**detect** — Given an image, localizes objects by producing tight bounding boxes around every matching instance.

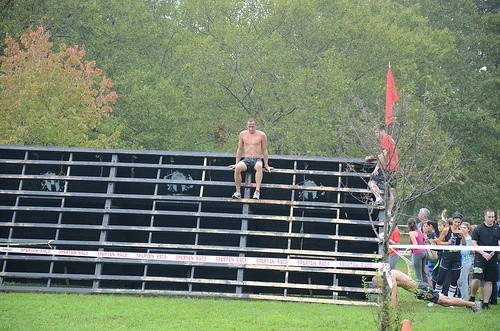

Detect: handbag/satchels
[431,251,438,263]
[424,242,432,259]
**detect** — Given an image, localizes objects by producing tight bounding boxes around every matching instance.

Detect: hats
[452,213,463,218]
[430,221,437,227]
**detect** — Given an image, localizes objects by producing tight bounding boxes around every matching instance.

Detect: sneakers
[253,190,260,199]
[232,192,241,199]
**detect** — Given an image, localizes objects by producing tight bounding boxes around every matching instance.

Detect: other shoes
[482,303,492,310]
[469,296,475,302]
[373,197,383,206]
[386,209,391,215]
[472,301,482,314]
[490,301,497,305]
[427,302,435,307]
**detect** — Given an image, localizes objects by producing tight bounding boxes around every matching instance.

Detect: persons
[228,119,274,200]
[372,208,500,313]
[366,123,398,216]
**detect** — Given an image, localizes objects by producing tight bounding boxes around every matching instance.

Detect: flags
[386,66,397,127]
